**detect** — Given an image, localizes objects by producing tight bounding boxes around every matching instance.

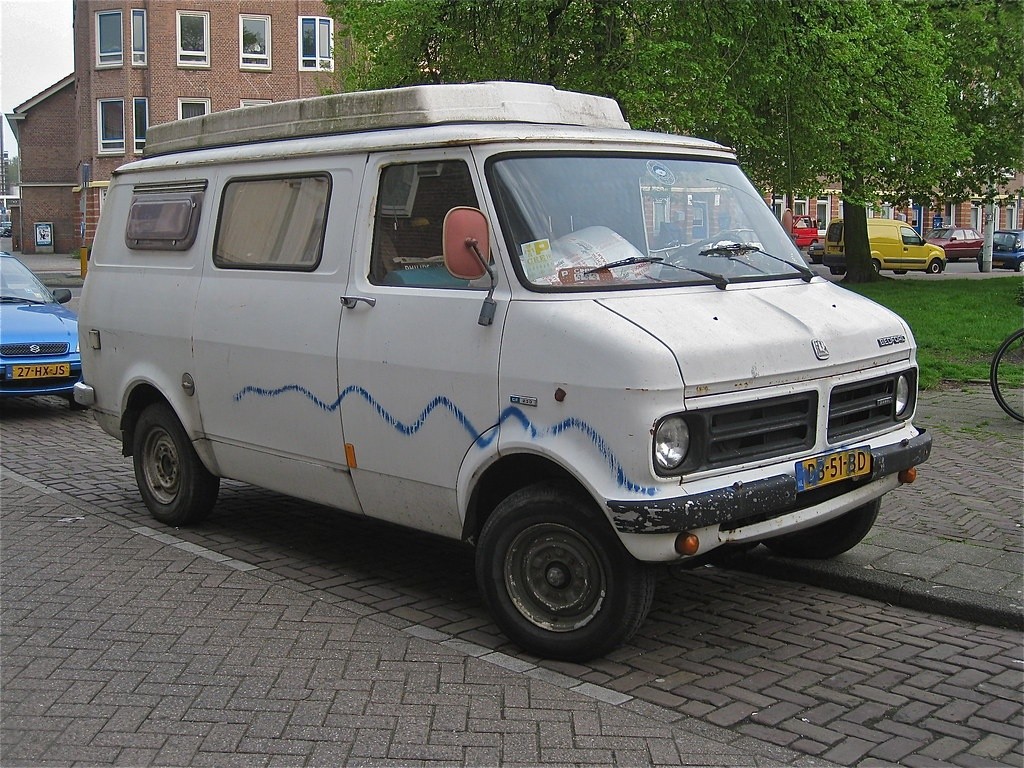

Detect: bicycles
[991,330,1024,422]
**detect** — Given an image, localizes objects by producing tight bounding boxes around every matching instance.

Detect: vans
[74,80,933,663]
[790,215,947,275]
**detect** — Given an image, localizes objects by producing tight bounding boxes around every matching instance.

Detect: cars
[923,228,984,262]
[977,230,1024,272]
[0,252,88,411]
